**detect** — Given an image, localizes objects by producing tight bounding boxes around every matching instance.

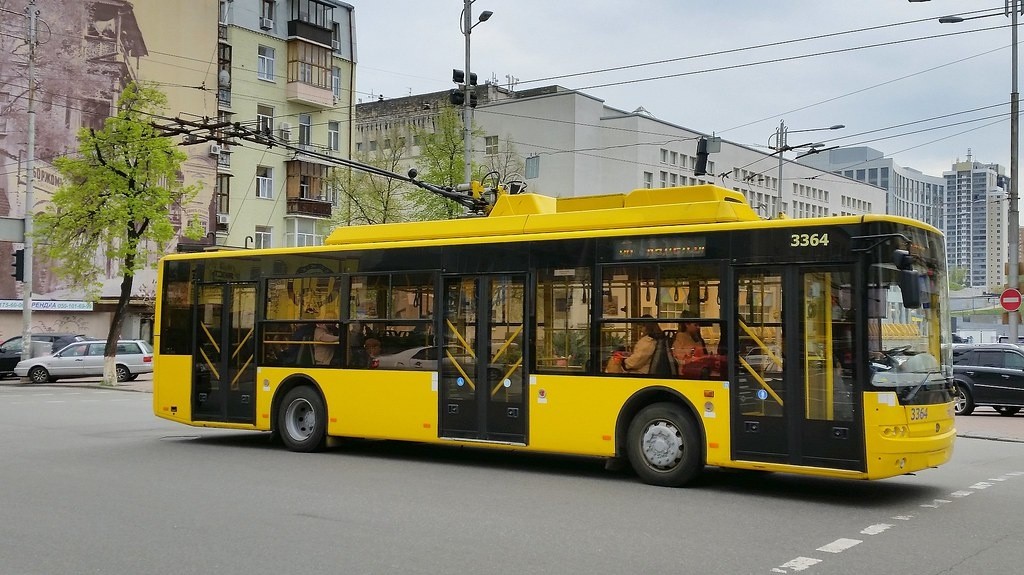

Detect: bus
[941,343,1024,416]
[152,122,958,481]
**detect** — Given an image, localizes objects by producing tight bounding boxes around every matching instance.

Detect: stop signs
[1000,288,1023,312]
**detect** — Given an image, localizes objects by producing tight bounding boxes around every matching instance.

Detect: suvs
[0,332,94,381]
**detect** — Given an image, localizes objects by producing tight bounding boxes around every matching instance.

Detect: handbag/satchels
[605,354,628,373]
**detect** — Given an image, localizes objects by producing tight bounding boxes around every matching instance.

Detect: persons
[406,315,430,345]
[615,314,662,373]
[272,308,371,363]
[838,311,884,379]
[359,340,382,368]
[670,309,760,378]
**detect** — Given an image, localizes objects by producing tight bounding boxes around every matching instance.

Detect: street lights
[938,0,1019,344]
[777,118,845,218]
[464,0,494,216]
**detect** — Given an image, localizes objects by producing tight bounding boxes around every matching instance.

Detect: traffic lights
[11,249,23,282]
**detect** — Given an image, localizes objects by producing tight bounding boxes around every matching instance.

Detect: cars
[14,339,153,384]
[953,335,1024,376]
[367,339,946,411]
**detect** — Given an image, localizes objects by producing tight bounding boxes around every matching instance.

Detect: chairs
[292,326,318,367]
[650,329,704,376]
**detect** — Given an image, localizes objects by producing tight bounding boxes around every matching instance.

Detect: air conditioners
[262,18,273,29]
[210,145,220,154]
[332,40,340,50]
[333,95,338,105]
[280,122,291,131]
[281,131,291,141]
[216,214,230,225]
